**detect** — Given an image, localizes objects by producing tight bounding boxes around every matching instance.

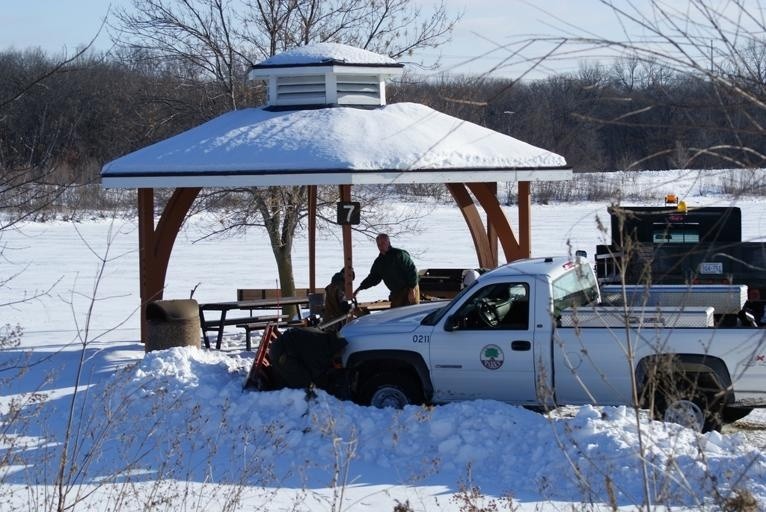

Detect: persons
[354,234,420,308]
[325,268,355,322]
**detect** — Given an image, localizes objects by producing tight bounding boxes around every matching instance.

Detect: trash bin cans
[145,300,202,352]
[309,292,324,320]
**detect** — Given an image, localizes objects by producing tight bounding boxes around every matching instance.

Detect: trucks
[574,205,766,300]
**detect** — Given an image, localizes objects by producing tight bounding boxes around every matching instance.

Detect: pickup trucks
[337,258,766,433]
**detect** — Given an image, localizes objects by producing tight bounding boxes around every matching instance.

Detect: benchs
[236,322,289,351]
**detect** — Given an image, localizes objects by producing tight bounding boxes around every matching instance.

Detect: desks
[200,299,310,348]
